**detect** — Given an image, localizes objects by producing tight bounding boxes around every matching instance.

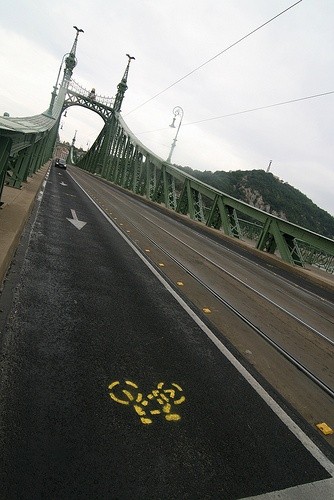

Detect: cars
[54,157,67,170]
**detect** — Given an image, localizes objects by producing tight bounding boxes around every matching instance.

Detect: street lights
[42,52,79,120]
[163,106,184,162]
